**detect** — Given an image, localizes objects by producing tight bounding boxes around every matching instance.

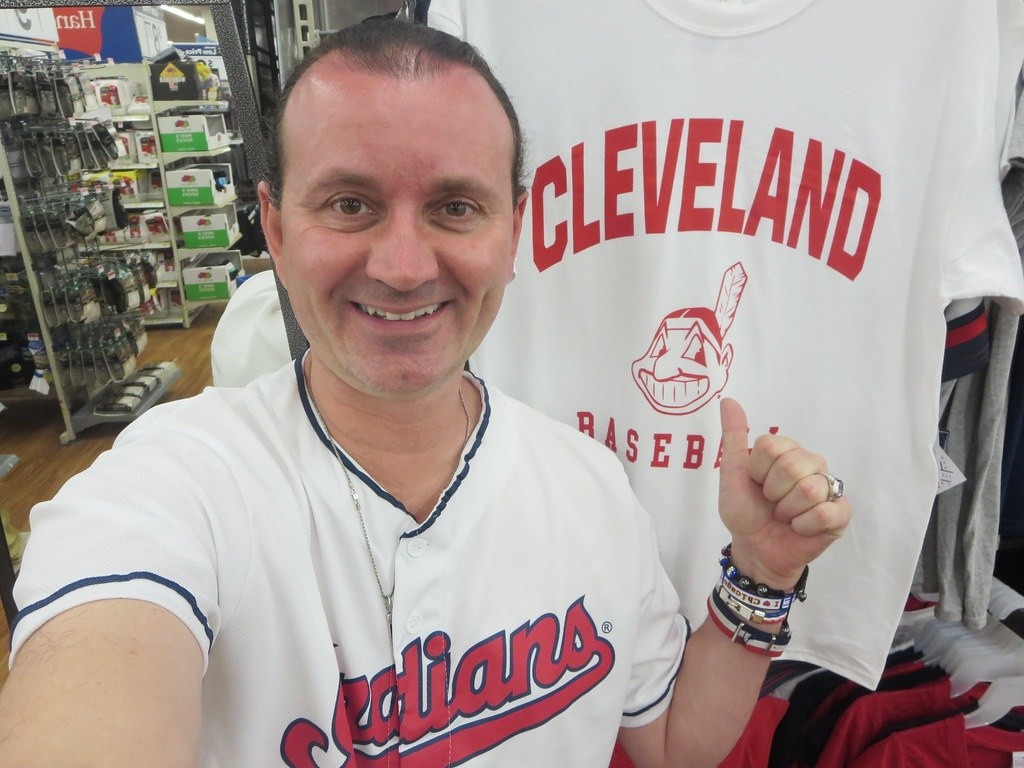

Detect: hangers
[898,575,1024,728]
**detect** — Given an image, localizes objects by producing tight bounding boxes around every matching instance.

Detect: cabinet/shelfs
[0,39,242,445]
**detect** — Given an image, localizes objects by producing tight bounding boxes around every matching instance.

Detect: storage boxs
[158,114,228,152]
[165,163,235,206]
[179,204,238,249]
[182,250,241,300]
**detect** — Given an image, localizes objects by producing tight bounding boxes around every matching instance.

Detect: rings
[816,472,844,502]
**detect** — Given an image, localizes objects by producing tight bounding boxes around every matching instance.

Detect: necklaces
[306,387,470,641]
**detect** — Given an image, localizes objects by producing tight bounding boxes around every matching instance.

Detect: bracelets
[707,543,809,658]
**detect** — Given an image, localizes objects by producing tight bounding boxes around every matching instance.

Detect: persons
[0,18,851,768]
